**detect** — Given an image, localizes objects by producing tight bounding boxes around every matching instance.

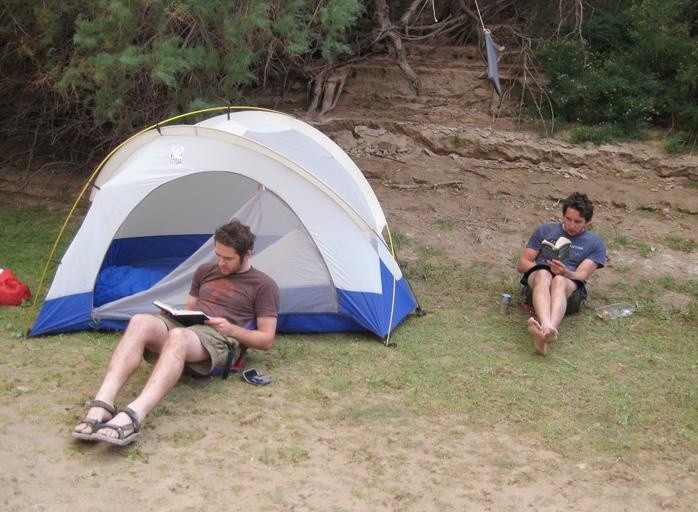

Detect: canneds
[499,293,511,314]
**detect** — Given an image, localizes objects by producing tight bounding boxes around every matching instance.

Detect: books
[540,237,572,262]
[151,301,210,327]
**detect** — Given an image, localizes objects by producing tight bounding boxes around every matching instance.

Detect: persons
[70,217,280,445]
[515,191,607,356]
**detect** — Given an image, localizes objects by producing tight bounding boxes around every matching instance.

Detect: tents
[21,103,430,353]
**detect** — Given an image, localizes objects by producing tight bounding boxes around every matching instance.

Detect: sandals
[72,400,118,442]
[96,407,142,446]
